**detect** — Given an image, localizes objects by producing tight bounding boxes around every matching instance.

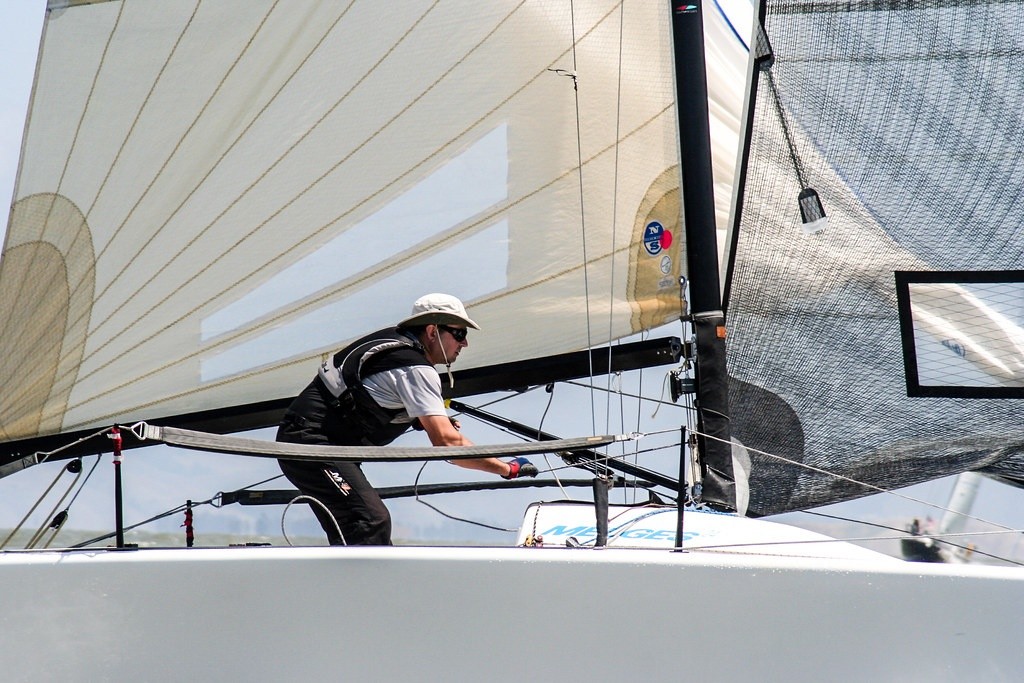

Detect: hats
[397,293,481,330]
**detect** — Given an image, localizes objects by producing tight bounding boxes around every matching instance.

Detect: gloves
[500,457,538,480]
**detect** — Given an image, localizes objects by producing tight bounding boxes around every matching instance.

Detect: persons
[273,289,538,550]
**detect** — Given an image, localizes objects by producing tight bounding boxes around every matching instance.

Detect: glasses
[438,324,467,342]
